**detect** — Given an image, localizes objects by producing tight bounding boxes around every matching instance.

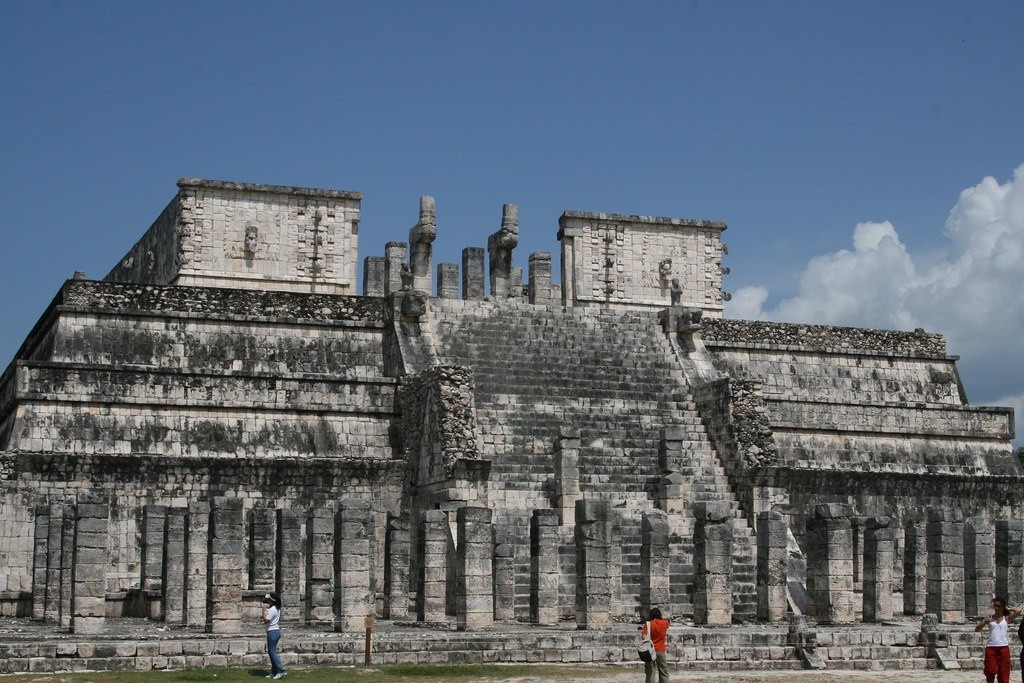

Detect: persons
[261,593,288,680]
[641,607,670,683]
[975,598,1021,683]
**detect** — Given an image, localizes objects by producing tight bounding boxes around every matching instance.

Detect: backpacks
[638,622,657,662]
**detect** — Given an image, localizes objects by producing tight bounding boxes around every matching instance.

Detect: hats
[265,594,277,602]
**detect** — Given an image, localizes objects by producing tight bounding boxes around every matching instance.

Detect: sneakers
[265,672,275,678]
[272,672,287,680]
